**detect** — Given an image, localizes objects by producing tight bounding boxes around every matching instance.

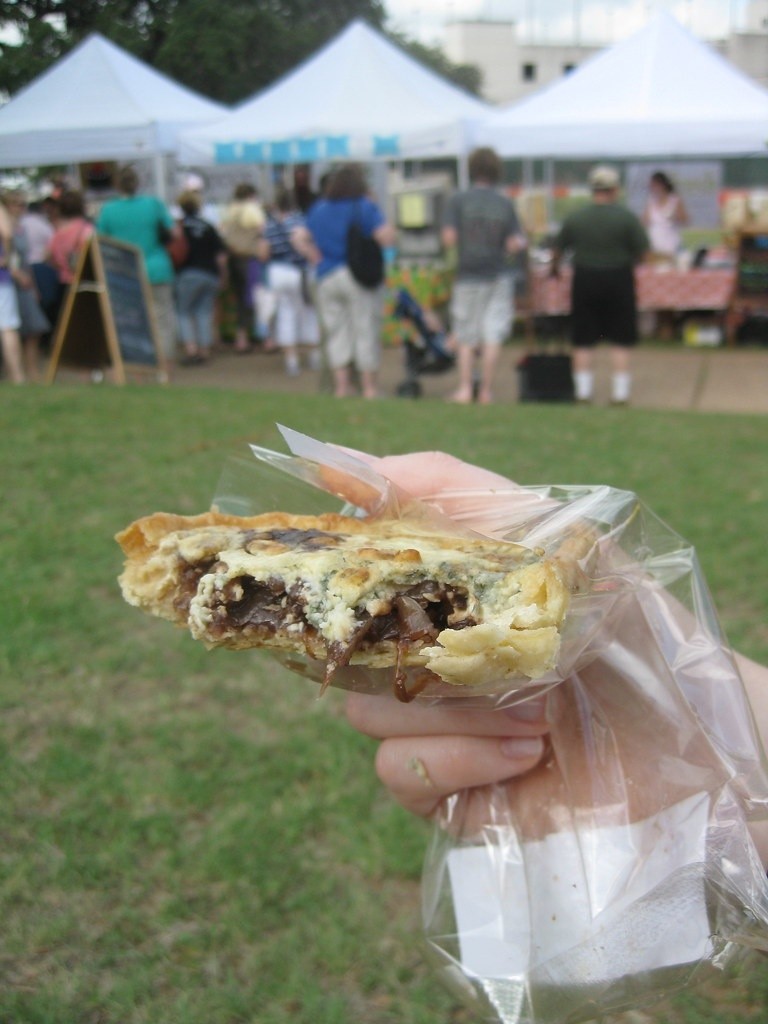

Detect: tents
[481,15,768,182]
[177,23,489,195]
[1,34,232,194]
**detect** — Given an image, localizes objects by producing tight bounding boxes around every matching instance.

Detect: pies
[104,510,566,700]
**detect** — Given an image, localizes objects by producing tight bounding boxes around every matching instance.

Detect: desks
[514,264,737,340]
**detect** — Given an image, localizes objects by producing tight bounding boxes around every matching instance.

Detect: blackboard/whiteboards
[53,235,169,371]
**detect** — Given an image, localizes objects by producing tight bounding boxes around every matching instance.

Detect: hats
[590,167,617,189]
[183,173,203,191]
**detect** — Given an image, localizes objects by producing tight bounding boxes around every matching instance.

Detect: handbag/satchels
[348,225,384,287]
[519,353,572,401]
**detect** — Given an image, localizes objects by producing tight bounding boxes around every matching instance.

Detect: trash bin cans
[517,352,579,402]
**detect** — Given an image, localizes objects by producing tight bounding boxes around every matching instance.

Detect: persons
[646,173,690,338]
[0,164,326,389]
[314,444,768,870]
[293,163,395,400]
[551,165,650,405]
[442,149,527,403]
